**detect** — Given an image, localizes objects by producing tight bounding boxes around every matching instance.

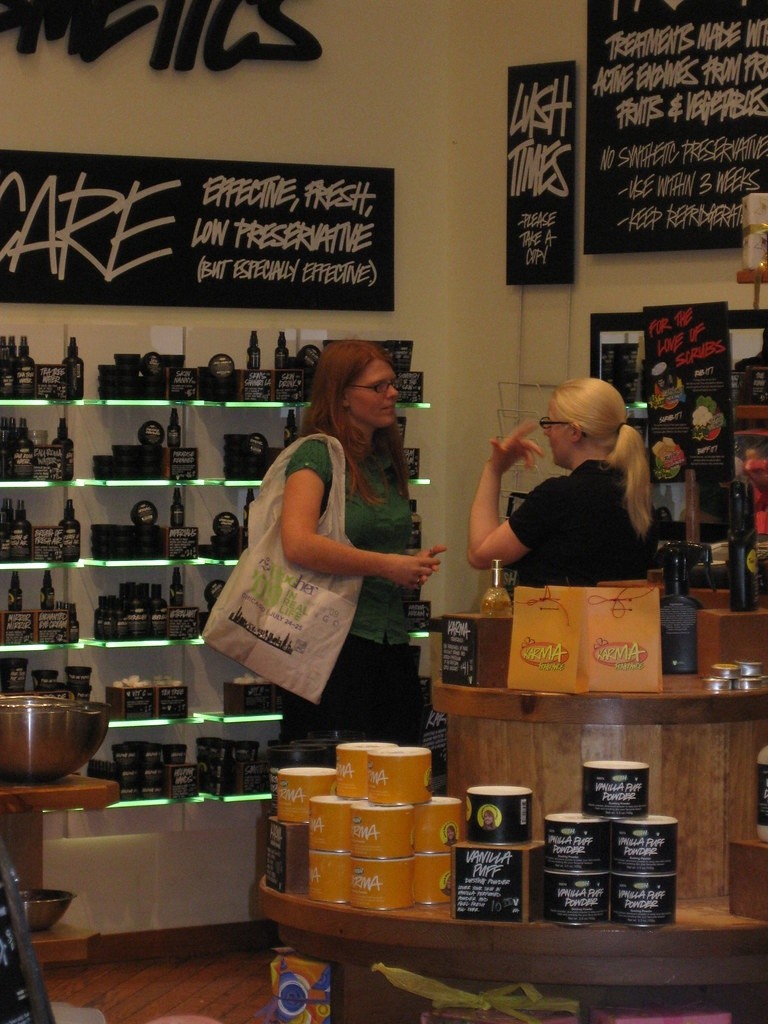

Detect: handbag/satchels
[202,435,364,703]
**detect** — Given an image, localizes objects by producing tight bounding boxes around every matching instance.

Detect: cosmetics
[0,330,449,800]
[442,539,715,694]
[728,479,759,612]
[757,744,768,842]
[700,658,768,691]
[265,740,679,927]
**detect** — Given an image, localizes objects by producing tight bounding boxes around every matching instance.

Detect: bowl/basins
[0,689,112,783]
[19,889,77,931]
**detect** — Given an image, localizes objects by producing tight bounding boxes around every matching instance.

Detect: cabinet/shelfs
[0,323,436,937]
[256,671,768,1024]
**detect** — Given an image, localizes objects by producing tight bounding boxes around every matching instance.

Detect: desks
[0,773,122,1024]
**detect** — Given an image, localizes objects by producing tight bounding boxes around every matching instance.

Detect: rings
[430,547,436,555]
[416,576,422,585]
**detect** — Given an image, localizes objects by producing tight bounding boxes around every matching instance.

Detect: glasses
[539,416,587,438]
[347,378,398,393]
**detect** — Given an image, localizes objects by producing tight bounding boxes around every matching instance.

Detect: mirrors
[587,310,768,544]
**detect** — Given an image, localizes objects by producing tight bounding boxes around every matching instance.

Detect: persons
[468,377,658,587]
[280,339,448,767]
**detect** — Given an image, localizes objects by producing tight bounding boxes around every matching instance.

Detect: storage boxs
[240,369,273,402]
[695,606,768,675]
[163,763,199,800]
[0,609,35,646]
[155,686,188,719]
[388,339,415,370]
[222,681,273,716]
[31,526,64,563]
[450,839,545,924]
[395,370,424,402]
[443,611,512,688]
[743,365,768,405]
[402,447,420,480]
[34,363,66,400]
[163,367,198,400]
[5,691,36,696]
[272,368,304,403]
[742,193,768,269]
[35,610,70,645]
[266,816,310,895]
[37,691,70,700]
[239,526,248,555]
[233,759,270,795]
[31,444,63,480]
[166,605,200,641]
[164,526,200,561]
[106,684,156,721]
[728,838,768,923]
[397,416,406,445]
[167,447,198,480]
